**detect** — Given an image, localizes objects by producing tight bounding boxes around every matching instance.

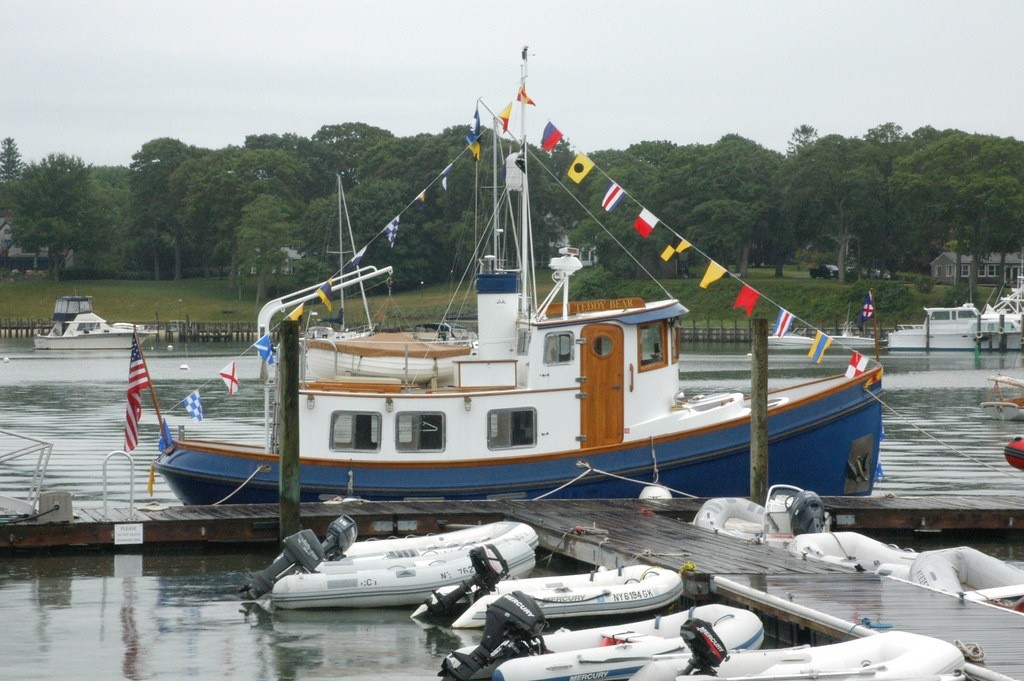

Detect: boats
[768,275,1024,349]
[409,545,968,681]
[156,45,886,506]
[33,288,158,349]
[687,484,1024,613]
[240,514,539,610]
[979,374,1024,471]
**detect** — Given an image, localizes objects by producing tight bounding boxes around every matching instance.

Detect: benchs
[601,629,664,643]
[964,583,1024,600]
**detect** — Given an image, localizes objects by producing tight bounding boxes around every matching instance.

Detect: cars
[810,264,890,281]
